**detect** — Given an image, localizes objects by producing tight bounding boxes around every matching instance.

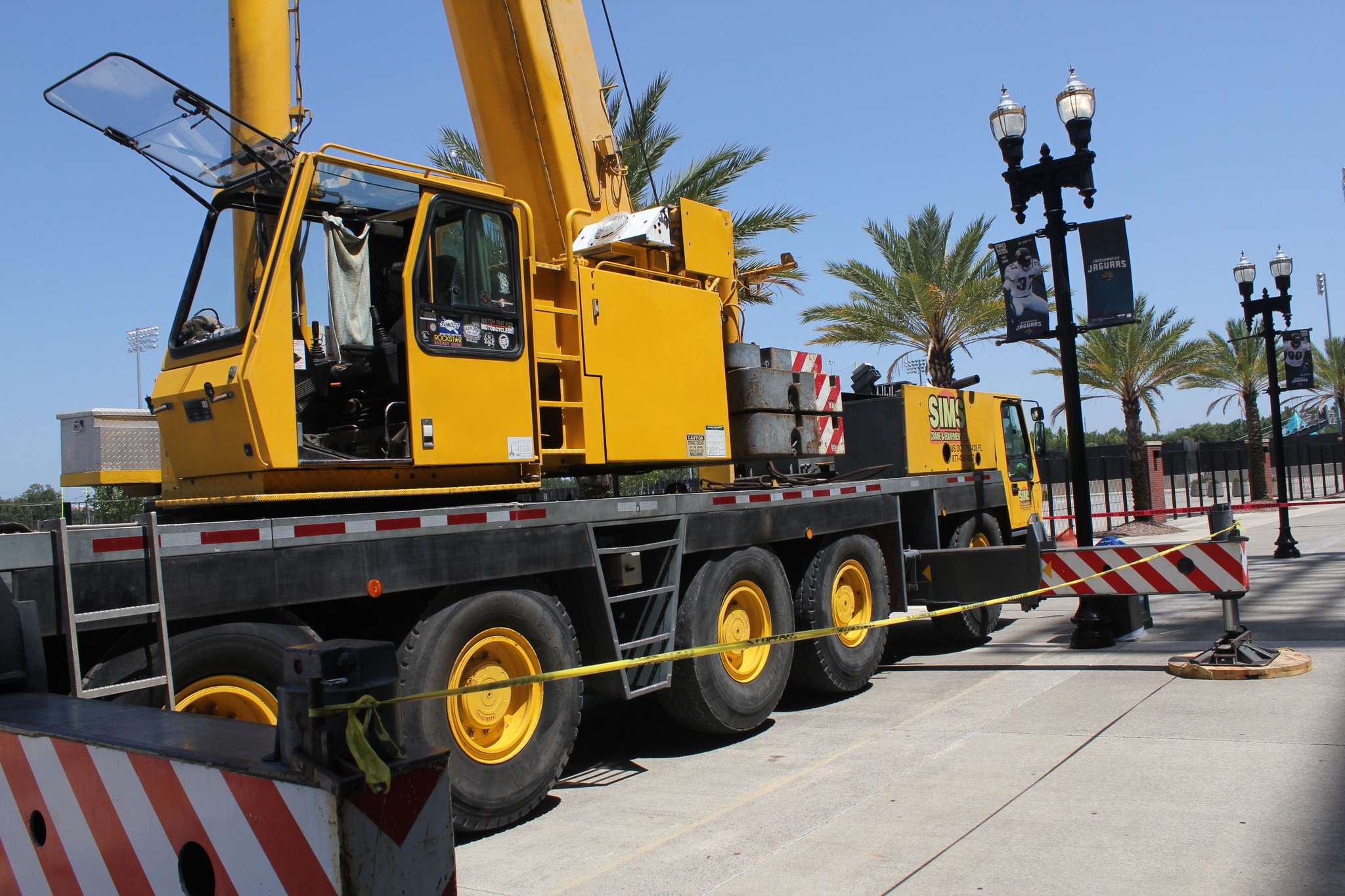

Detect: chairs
[420,255,463,308]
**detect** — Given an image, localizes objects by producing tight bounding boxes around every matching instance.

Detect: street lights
[1316,271,1340,390]
[1230,242,1302,559]
[988,65,1117,651]
[126,325,160,410]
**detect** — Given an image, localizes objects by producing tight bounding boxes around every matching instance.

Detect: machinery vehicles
[1,0,1316,896]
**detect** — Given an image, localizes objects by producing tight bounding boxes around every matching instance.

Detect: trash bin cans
[1095,537,1154,638]
